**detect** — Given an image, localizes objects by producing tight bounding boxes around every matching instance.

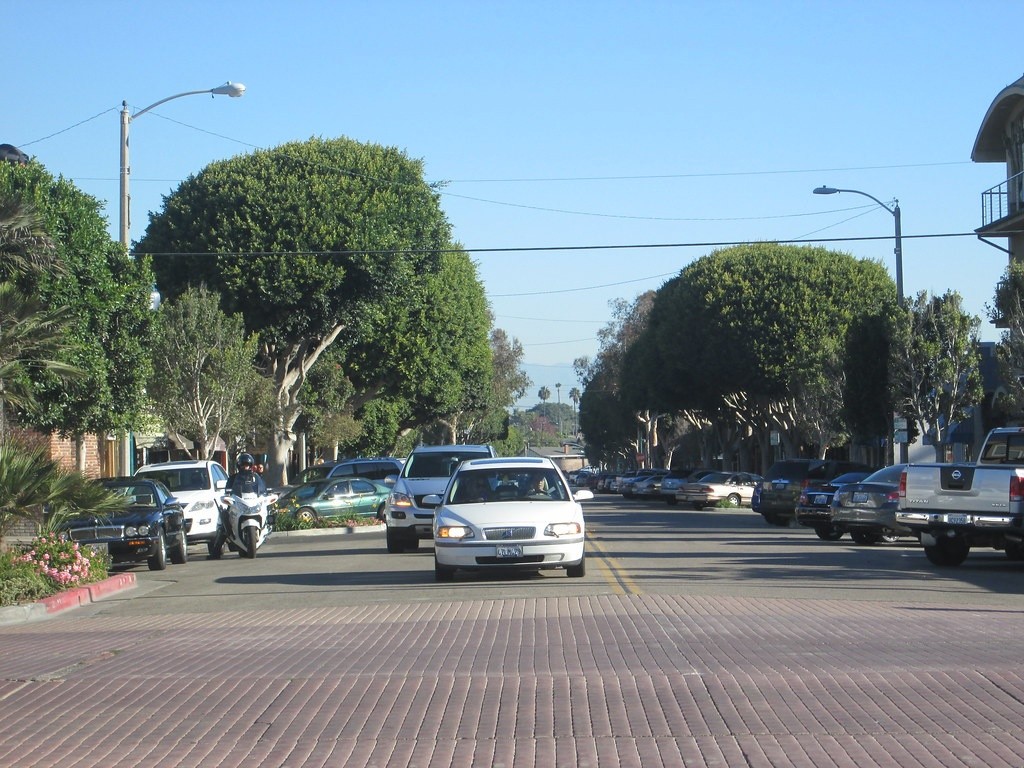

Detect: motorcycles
[219,480,280,559]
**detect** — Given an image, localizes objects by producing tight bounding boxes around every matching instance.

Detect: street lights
[813,184,909,464]
[118,80,248,476]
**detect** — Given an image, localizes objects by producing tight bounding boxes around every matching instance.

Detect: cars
[795,463,901,544]
[273,476,392,532]
[675,471,763,511]
[829,463,922,547]
[568,464,714,506]
[421,456,596,583]
[43,478,189,580]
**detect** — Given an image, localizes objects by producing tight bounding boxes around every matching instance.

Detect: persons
[204,450,267,560]
[523,471,555,498]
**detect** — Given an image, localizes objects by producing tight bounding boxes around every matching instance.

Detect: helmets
[237,452,254,474]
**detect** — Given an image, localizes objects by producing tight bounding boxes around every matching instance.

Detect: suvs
[384,444,501,553]
[277,456,403,498]
[751,458,863,526]
[121,459,230,556]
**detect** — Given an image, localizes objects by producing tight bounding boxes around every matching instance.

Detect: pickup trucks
[895,425,1024,565]
